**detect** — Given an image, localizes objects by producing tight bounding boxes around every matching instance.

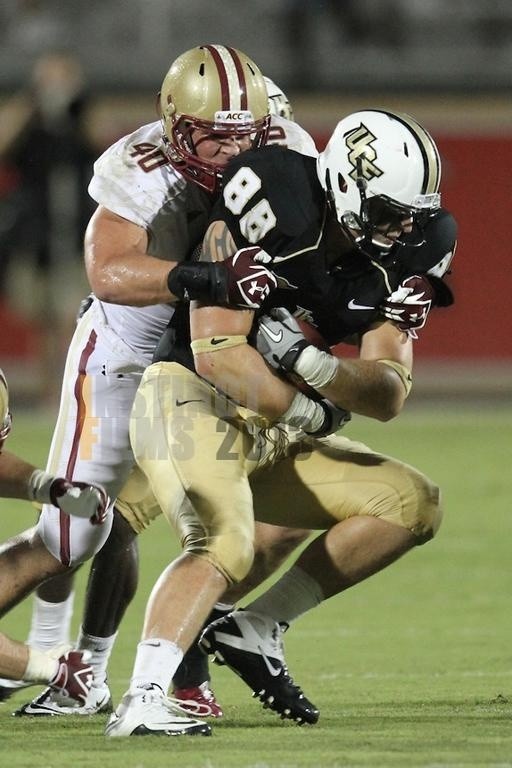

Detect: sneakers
[0,672,112,716]
[104,678,226,738]
[197,607,320,725]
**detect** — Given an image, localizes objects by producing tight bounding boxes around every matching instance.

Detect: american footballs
[256,310,334,401]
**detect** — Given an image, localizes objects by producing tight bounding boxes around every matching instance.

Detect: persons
[1,38,462,736]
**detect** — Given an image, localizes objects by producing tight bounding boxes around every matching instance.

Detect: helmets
[0,368,13,451]
[316,107,442,270]
[156,44,294,196]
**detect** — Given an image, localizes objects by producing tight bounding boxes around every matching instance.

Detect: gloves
[46,648,94,707]
[50,477,110,526]
[248,304,310,374]
[377,273,436,332]
[209,244,278,310]
